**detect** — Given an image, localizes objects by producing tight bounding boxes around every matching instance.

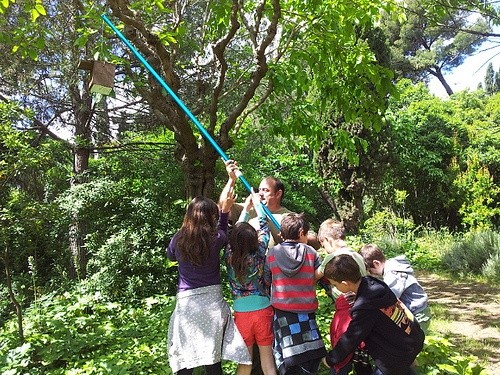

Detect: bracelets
[267,244,270,252]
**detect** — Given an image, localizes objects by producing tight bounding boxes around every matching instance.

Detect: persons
[357,243,431,375]
[167,187,252,375]
[218,156,321,256]
[224,194,275,375]
[315,218,373,375]
[324,254,425,375]
[264,213,324,375]
